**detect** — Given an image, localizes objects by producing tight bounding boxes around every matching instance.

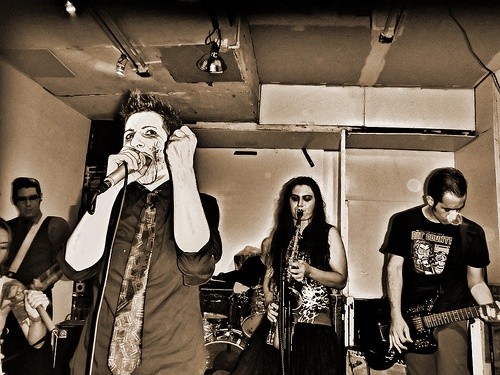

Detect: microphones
[295,207,305,227]
[96,154,146,193]
[26,290,56,335]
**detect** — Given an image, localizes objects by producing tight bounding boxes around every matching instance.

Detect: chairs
[53,294,93,368]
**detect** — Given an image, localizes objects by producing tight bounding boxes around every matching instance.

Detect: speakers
[199,288,241,333]
[344,350,409,375]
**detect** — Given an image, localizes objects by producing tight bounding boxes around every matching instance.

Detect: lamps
[115,54,128,76]
[196,17,227,74]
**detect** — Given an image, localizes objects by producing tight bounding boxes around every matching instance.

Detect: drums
[329,294,345,348]
[202,313,229,330]
[237,284,266,338]
[203,325,249,374]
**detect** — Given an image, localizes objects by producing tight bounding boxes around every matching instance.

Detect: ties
[107,192,159,375]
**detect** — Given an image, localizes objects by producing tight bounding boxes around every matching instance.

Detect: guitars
[359,298,500,371]
[28,263,64,295]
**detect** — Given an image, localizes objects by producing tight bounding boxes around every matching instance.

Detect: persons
[378,166,500,375]
[233,176,347,374]
[0,176,76,375]
[230,236,271,339]
[64,89,222,375]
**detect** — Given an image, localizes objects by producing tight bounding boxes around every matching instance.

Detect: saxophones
[265,209,304,354]
